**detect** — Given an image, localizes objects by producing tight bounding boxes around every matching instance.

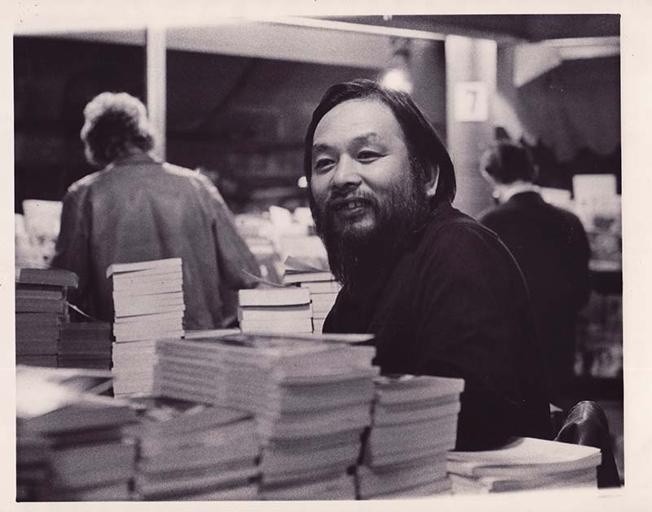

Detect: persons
[50,87,258,332]
[466,136,591,400]
[303,71,552,453]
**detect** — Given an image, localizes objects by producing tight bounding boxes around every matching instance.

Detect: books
[15,254,606,503]
